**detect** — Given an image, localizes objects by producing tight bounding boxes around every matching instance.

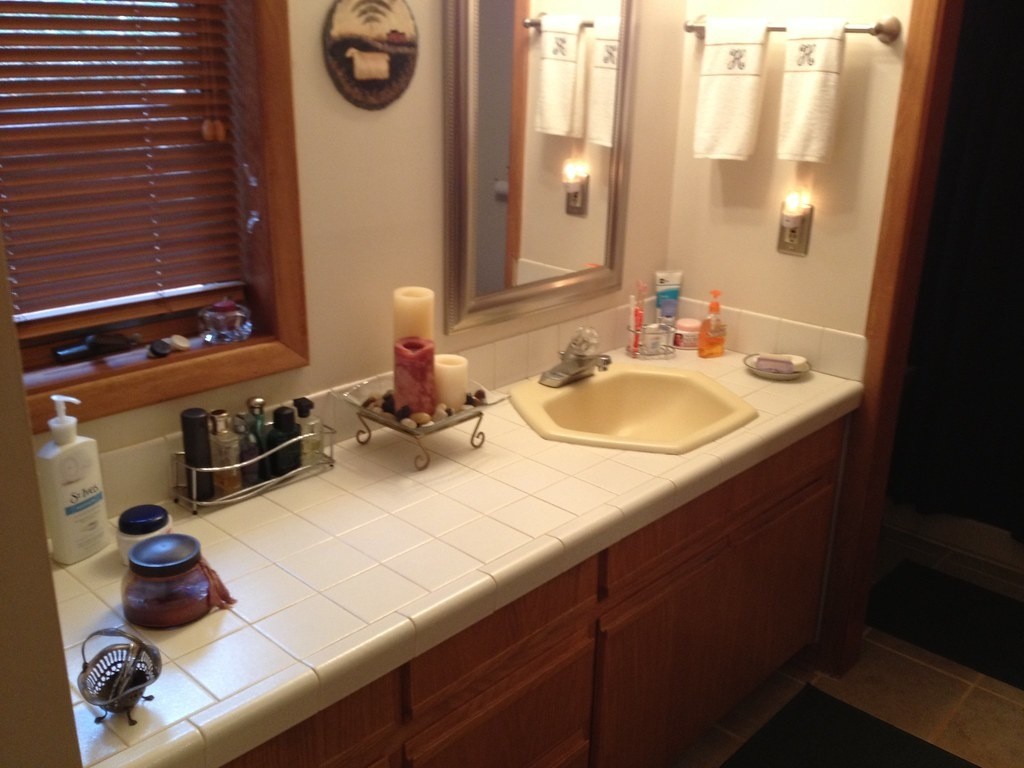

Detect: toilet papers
[493,179,509,195]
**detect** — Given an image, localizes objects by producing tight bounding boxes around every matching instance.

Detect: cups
[434,354,468,412]
[394,286,433,341]
[392,336,435,416]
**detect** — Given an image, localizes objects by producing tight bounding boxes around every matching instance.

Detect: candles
[435,352,468,411]
[393,336,435,416]
[394,286,433,341]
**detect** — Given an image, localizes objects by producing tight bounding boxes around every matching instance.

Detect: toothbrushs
[631,278,647,358]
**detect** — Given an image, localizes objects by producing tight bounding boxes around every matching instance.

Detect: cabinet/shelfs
[221,413,854,768]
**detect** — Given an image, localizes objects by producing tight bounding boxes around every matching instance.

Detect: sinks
[508,361,761,455]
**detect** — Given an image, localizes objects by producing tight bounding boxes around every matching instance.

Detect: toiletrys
[35,395,110,565]
[655,270,684,328]
[121,533,236,628]
[180,396,323,502]
[698,289,724,359]
[116,505,173,567]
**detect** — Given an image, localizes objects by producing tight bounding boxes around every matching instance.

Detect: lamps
[564,157,590,191]
[781,184,812,227]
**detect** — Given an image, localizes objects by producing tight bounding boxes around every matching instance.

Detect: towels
[777,19,849,165]
[690,16,769,164]
[535,15,585,139]
[587,16,621,148]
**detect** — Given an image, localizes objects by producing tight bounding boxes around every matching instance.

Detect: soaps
[757,354,807,373]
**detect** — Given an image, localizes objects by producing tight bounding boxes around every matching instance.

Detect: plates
[742,354,812,380]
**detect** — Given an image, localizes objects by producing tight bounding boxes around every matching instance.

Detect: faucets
[539,326,612,388]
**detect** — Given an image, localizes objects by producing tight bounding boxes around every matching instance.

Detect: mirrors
[440,0,641,338]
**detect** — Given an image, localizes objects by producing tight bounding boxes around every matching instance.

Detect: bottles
[119,534,214,628]
[182,396,322,500]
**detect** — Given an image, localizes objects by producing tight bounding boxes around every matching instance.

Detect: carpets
[715,678,983,768]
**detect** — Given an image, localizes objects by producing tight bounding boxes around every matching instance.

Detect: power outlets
[561,175,590,216]
[776,205,814,255]
